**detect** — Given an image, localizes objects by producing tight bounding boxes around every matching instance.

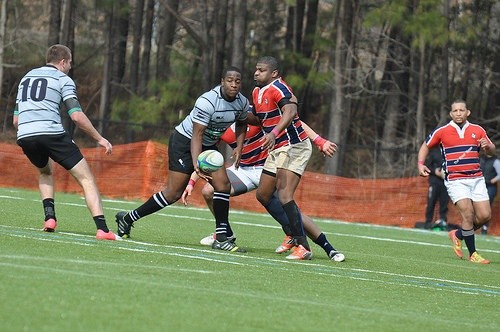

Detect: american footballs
[196,149,224,173]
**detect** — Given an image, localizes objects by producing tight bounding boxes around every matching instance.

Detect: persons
[247,54,316,261]
[417,98,496,265]
[114,66,250,254]
[423,144,450,230]
[180,104,346,263]
[12,44,125,241]
[479,147,500,239]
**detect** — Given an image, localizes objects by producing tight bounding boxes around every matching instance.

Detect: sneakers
[448,229,463,258]
[327,250,346,262]
[96,228,123,242]
[286,243,313,261]
[275,235,296,254]
[467,251,489,264]
[43,219,57,232]
[115,211,135,239]
[200,233,218,246]
[212,236,247,253]
